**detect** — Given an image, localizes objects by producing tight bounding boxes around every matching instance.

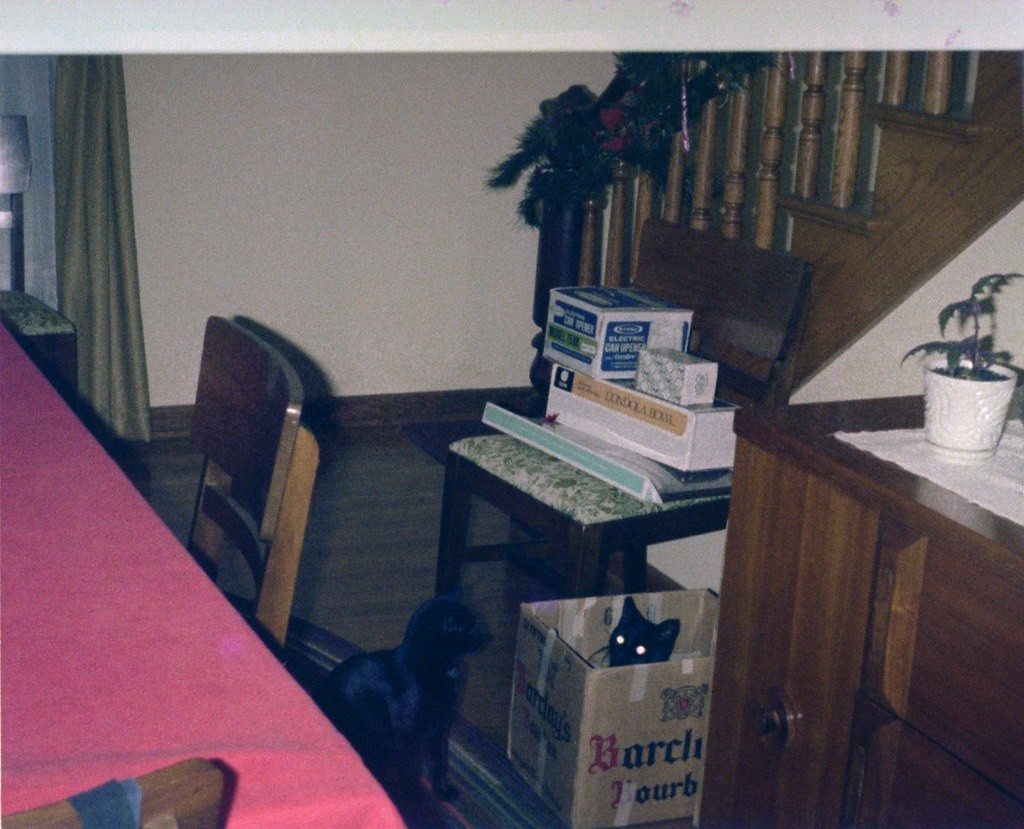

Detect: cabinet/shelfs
[693,434,1023,829]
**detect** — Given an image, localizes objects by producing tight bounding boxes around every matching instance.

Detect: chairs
[0,114,77,391]
[183,312,317,666]
[433,217,817,620]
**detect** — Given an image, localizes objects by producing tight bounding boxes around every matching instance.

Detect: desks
[0,318,409,829]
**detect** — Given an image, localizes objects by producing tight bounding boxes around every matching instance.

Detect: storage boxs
[541,286,696,384]
[633,347,719,407]
[508,587,725,828]
[544,363,738,472]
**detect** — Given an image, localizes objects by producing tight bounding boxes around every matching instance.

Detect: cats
[585,596,681,668]
[319,588,479,807]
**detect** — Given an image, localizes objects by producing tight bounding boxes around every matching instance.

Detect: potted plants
[900,272,1024,464]
